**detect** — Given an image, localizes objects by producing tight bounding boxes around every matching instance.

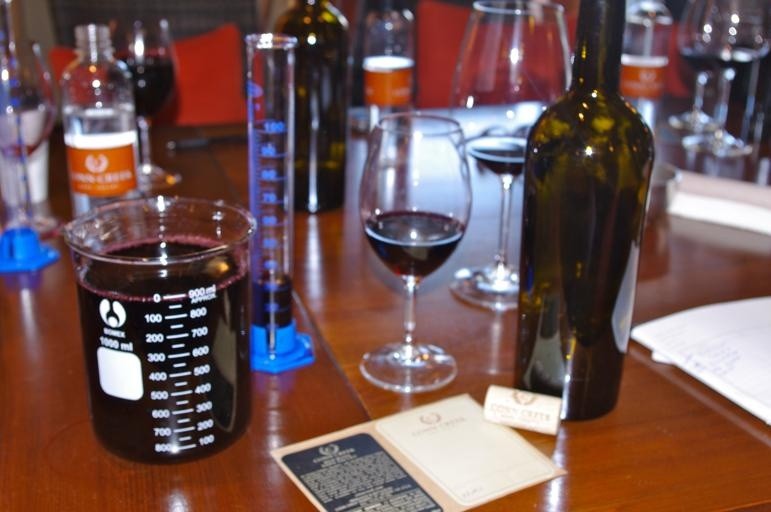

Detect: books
[642,162,771,238]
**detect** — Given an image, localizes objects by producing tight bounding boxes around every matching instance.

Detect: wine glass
[442,0,571,314]
[356,112,474,396]
[668,0,770,163]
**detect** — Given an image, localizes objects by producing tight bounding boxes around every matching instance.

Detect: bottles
[511,0,657,424]
[0,2,422,375]
[620,0,672,150]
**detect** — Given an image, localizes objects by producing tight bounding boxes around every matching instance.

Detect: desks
[2,98,771,510]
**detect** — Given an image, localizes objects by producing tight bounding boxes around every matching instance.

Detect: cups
[59,195,256,468]
